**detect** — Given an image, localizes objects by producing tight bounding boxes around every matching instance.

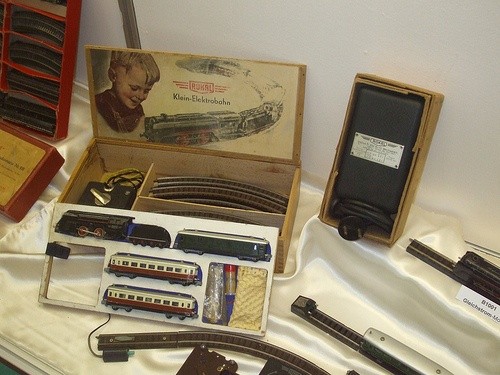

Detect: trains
[360,327,454,375]
[53,209,269,321]
[452,251,500,305]
